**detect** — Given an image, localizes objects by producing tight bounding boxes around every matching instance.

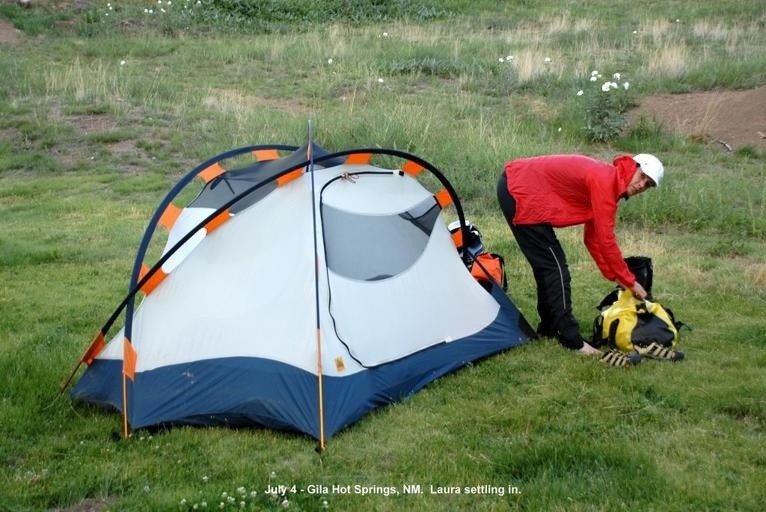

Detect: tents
[58,121,541,450]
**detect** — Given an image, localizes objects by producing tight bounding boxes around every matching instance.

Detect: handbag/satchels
[623,256,653,300]
[593,288,689,351]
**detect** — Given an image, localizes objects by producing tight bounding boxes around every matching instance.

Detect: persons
[498,152,665,358]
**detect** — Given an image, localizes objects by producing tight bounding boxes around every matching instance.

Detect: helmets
[632,153,664,189]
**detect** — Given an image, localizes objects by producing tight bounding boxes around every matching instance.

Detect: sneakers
[634,343,685,361]
[599,350,641,367]
